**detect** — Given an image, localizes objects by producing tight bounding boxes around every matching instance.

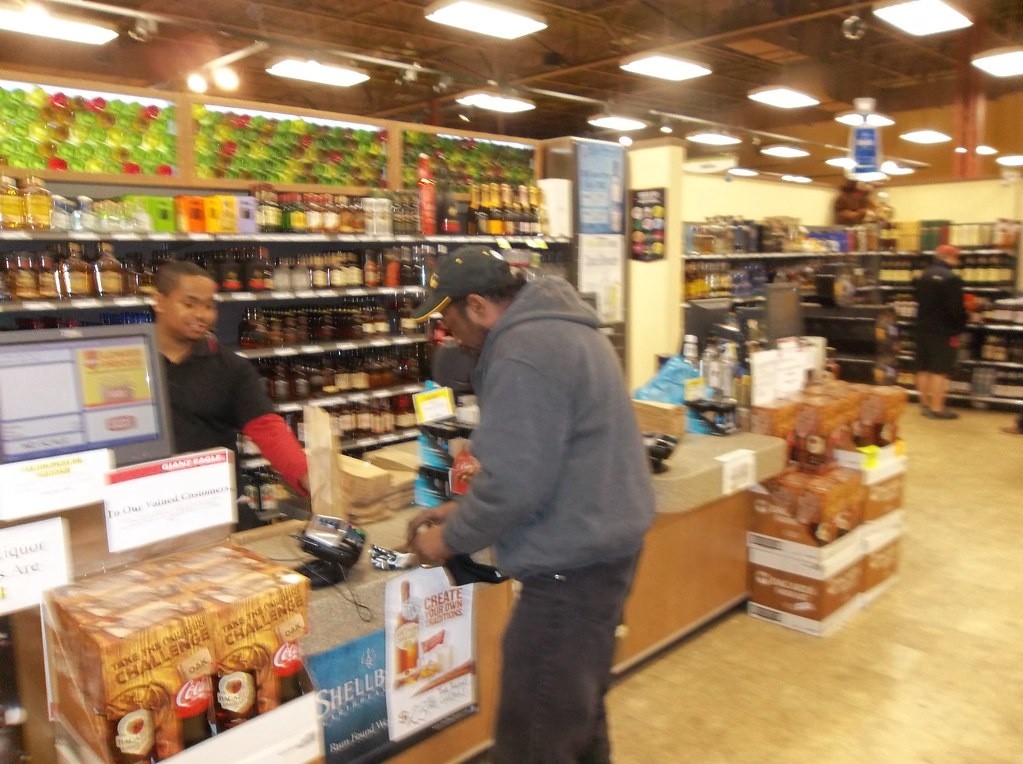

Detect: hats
[411,246,506,323]
[936,245,958,266]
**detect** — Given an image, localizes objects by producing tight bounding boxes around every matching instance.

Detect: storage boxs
[40,540,331,764]
[751,379,911,643]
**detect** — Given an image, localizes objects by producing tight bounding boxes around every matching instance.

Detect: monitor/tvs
[0,324,174,470]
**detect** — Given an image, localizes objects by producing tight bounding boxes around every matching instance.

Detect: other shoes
[928,409,958,419]
[919,404,929,415]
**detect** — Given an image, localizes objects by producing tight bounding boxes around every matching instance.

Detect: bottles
[17,311,153,331]
[894,360,915,389]
[0,240,178,301]
[685,261,767,300]
[0,173,153,234]
[280,392,417,447]
[251,345,433,402]
[251,178,549,237]
[964,368,1023,400]
[242,468,286,512]
[693,214,735,255]
[239,293,442,348]
[784,261,823,289]
[892,293,918,320]
[181,243,448,291]
[682,334,759,406]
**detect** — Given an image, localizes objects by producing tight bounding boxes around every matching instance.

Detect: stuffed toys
[834,180,877,224]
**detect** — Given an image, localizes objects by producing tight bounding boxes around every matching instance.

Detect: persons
[914,245,968,420]
[148,260,311,533]
[405,245,654,764]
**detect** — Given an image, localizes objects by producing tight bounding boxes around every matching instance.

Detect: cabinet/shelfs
[683,252,1023,401]
[0,69,571,467]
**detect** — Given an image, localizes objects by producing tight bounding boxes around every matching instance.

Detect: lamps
[0,0,120,46]
[266,51,372,86]
[456,89,537,114]
[425,0,549,40]
[619,50,714,82]
[587,104,653,131]
[759,139,811,158]
[969,39,1023,78]
[871,0,976,36]
[685,125,743,146]
[747,85,821,109]
